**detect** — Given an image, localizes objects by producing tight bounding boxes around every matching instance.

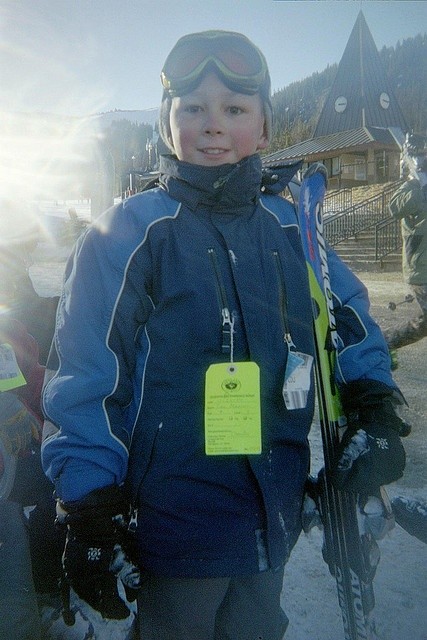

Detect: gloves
[333,379,412,494]
[54,484,142,619]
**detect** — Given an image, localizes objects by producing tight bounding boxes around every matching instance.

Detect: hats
[158,28,274,154]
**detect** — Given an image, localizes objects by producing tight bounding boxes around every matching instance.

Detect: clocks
[334,96,348,113]
[379,91,391,110]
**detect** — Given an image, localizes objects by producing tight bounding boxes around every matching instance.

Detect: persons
[388,130,427,324]
[39,28,412,640]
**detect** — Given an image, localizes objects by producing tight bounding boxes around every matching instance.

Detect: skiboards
[287,162,382,640]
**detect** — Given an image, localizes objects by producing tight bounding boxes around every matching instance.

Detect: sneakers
[392,497,426,542]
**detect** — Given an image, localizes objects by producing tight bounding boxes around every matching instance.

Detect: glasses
[160,32,267,97]
